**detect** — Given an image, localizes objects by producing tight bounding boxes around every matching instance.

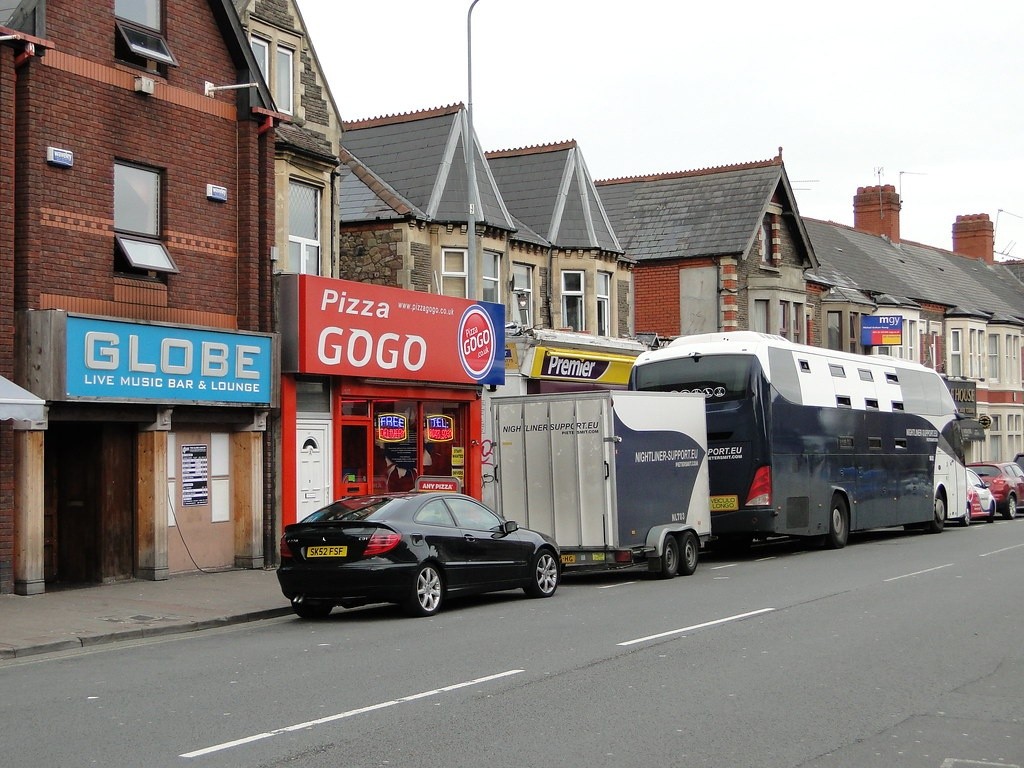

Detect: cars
[964,452,1024,520]
[966,467,996,523]
[276,491,563,622]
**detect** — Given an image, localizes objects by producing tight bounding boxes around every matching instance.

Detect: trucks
[488,391,719,578]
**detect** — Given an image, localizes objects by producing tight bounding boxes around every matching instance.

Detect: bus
[627,330,968,552]
